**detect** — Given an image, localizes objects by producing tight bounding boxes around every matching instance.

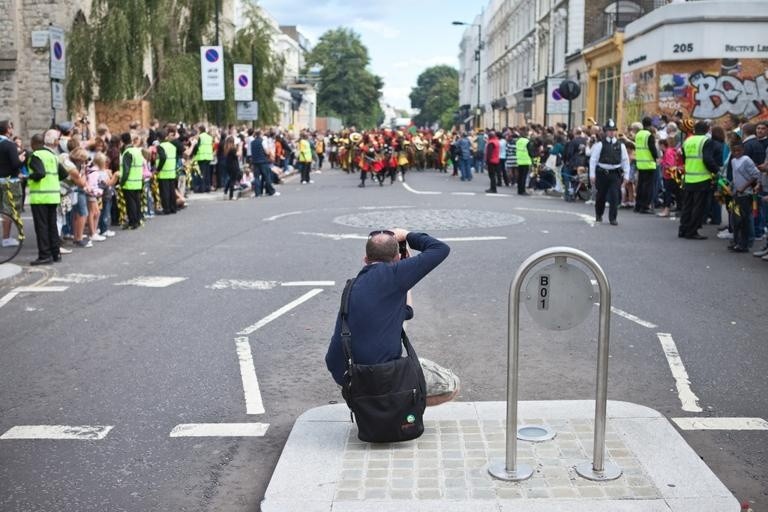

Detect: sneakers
[634,189,768,262]
[192,176,318,201]
[1,237,24,247]
[64,227,116,247]
[59,246,72,253]
[484,177,633,227]
[29,253,62,265]
[319,162,483,188]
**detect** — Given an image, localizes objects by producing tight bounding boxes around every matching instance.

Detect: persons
[326,228,460,412]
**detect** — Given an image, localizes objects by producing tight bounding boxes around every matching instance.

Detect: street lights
[452,20,483,131]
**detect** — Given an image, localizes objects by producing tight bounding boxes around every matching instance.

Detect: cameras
[398,238,407,260]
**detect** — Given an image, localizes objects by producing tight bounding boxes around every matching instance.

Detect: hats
[678,117,696,130]
[57,122,73,133]
[349,132,364,143]
[604,117,618,130]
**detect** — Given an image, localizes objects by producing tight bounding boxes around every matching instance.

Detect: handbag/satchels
[339,276,428,443]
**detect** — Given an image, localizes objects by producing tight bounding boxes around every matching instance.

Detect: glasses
[368,229,395,239]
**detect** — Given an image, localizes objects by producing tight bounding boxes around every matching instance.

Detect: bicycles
[0,209,23,265]
